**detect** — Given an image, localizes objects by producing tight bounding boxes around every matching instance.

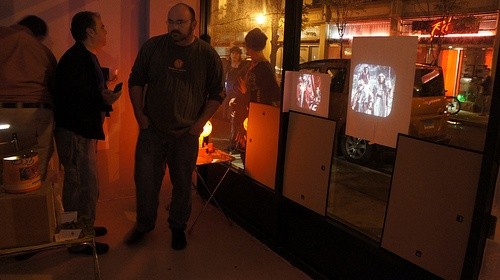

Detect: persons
[296,68,390,118]
[220,27,279,169]
[481,69,492,117]
[119,3,226,253]
[0,11,124,256]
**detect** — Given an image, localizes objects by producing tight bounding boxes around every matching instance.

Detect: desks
[187,148,236,233]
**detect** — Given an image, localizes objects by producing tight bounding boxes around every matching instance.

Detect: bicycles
[445,90,461,115]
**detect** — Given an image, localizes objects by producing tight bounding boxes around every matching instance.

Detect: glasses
[165,19,193,26]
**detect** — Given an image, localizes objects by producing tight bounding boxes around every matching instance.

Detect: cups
[200,147,209,156]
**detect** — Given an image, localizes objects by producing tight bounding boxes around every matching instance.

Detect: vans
[299,58,449,165]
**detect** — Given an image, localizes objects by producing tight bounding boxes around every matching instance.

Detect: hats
[229,46,242,54]
[245,28,268,51]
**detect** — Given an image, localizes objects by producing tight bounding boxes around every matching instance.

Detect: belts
[0,101,52,110]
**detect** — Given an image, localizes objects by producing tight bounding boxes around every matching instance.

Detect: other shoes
[122,224,157,245]
[170,226,188,252]
[66,240,110,257]
[93,226,108,238]
